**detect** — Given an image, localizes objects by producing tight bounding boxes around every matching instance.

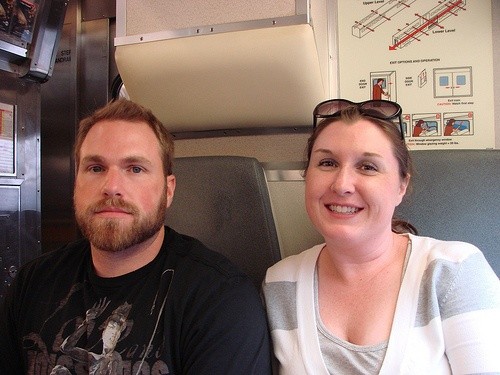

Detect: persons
[261,108,500,375]
[0,97,275,375]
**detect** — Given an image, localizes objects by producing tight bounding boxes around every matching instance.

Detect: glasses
[313,99,405,145]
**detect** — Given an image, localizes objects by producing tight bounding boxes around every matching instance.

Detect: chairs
[163,156,282,285]
[393,148,500,281]
